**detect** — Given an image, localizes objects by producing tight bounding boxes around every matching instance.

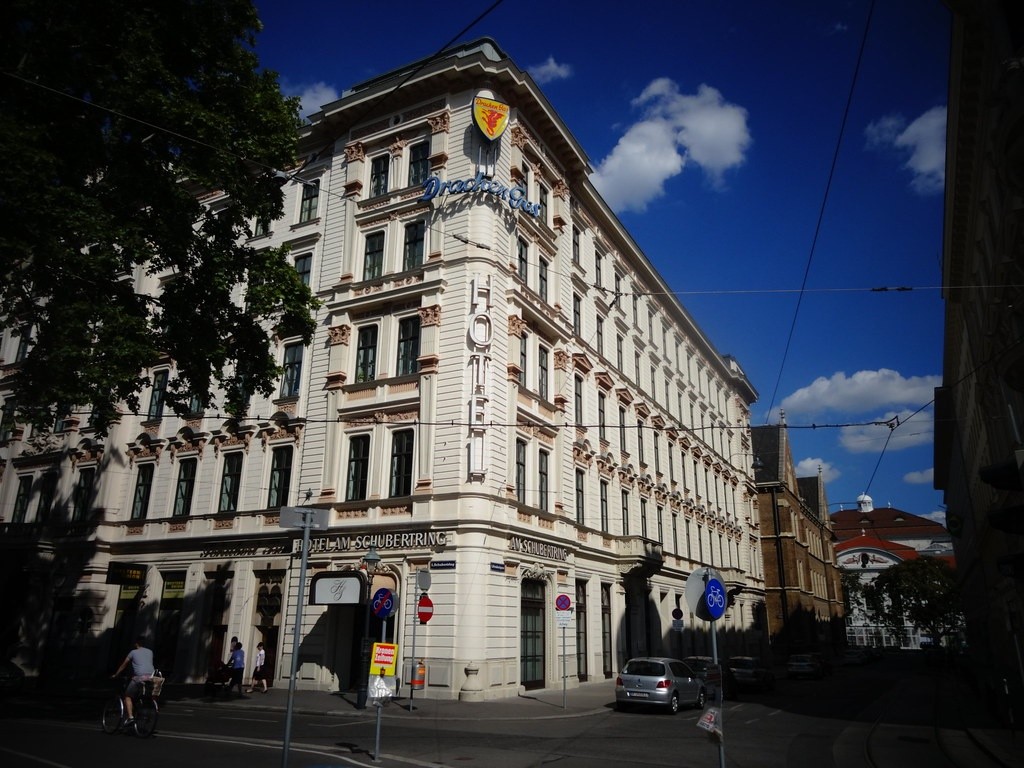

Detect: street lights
[356,545,381,708]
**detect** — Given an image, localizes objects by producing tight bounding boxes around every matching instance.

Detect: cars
[721,656,773,693]
[0,656,25,692]
[683,656,737,700]
[783,654,822,680]
[615,657,708,716]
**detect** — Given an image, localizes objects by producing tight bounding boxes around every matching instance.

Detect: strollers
[204,663,233,696]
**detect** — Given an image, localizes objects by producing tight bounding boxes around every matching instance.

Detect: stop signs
[418,597,434,622]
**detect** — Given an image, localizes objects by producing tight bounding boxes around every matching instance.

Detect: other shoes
[123,717,135,726]
[261,690,268,693]
[245,689,253,693]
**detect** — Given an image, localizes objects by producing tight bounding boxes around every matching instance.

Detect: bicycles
[102,676,160,739]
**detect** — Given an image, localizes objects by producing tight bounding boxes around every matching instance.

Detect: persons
[112,640,154,726]
[228,643,244,697]
[946,649,956,669]
[245,642,268,693]
[960,648,971,671]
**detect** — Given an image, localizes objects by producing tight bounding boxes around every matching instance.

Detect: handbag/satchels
[139,669,165,695]
